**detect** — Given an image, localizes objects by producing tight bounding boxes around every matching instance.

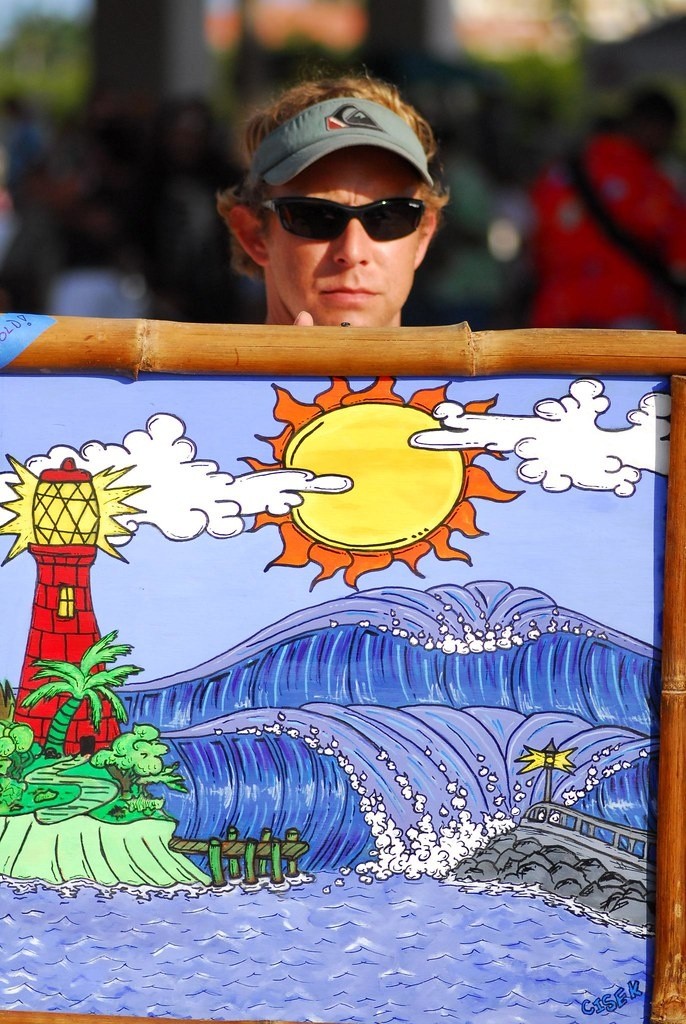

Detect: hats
[249,97,434,186]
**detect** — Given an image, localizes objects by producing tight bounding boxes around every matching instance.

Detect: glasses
[258,197,426,241]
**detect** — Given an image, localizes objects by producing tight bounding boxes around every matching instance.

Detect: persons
[0,91,234,322]
[529,88,686,330]
[217,75,451,328]
